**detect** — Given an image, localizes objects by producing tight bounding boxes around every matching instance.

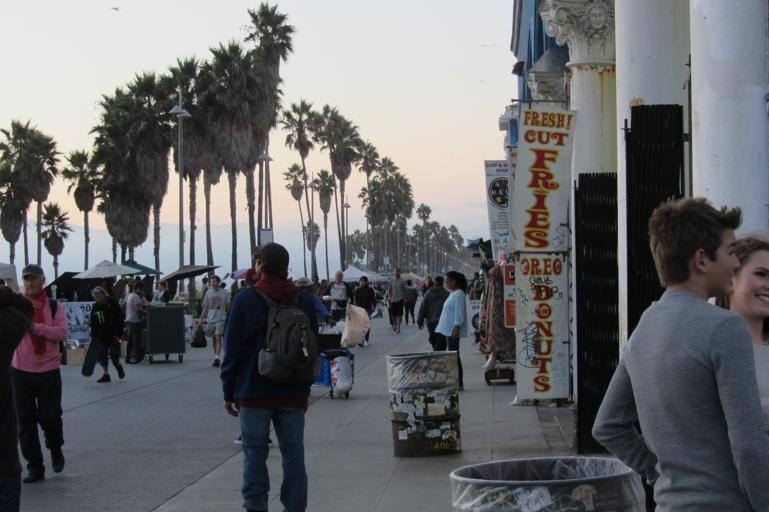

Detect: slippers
[251,285,320,383]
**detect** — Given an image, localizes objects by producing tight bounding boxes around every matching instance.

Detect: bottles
[22,264,43,278]
[296,277,311,286]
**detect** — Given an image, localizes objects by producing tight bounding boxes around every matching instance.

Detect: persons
[713,230,769,435]
[123,275,232,368]
[383,266,479,351]
[590,192,768,511]
[231,274,329,298]
[8,265,68,482]
[355,276,376,346]
[435,272,467,391]
[88,287,125,383]
[1,280,34,509]
[216,242,321,512]
[322,271,353,323]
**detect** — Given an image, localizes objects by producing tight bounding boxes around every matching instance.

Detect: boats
[145,305,186,357]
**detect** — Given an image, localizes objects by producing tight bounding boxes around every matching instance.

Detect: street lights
[191,326,207,348]
[334,300,347,308]
[331,357,352,395]
[340,303,370,348]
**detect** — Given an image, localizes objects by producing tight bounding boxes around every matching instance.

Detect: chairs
[97,375,110,382]
[212,359,220,367]
[117,364,124,378]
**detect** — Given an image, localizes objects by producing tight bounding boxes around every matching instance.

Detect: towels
[386,350,462,457]
[449,456,635,512]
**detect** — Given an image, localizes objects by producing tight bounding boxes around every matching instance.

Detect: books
[63,348,84,366]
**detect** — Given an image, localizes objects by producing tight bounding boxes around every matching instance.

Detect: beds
[157,264,222,284]
[335,265,426,286]
[121,259,163,277]
[228,267,255,281]
[70,258,144,282]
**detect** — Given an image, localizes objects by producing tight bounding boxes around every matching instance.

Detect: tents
[335,265,426,286]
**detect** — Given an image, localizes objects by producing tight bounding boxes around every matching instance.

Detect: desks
[340,199,351,268]
[170,104,194,268]
[258,153,275,245]
[365,213,478,277]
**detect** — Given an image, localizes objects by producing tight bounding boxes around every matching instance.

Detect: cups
[93,288,101,294]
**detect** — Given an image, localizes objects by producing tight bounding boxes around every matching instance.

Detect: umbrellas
[51,448,65,473]
[23,473,44,483]
